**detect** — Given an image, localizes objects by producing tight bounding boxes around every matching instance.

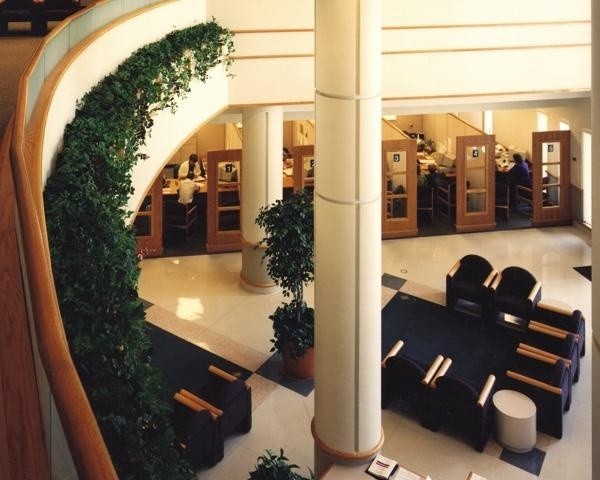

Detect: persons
[177,171,201,208]
[507,153,531,187]
[417,165,429,187]
[427,162,448,190]
[177,153,202,178]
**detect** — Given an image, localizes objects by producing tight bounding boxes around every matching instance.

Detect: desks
[416,151,457,179]
[492,389,537,452]
[162,156,241,220]
[283,159,314,190]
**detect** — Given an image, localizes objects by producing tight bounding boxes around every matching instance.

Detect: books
[192,176,205,183]
[365,454,399,480]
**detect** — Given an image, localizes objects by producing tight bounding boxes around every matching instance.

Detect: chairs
[432,355,496,452]
[382,339,445,410]
[529,309,583,359]
[164,200,199,241]
[171,363,252,470]
[417,183,469,226]
[445,254,498,324]
[504,347,572,440]
[495,175,550,221]
[518,324,581,408]
[490,265,543,336]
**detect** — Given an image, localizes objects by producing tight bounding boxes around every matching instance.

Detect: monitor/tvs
[436,147,456,168]
[507,145,527,162]
[218,163,237,181]
[304,160,313,177]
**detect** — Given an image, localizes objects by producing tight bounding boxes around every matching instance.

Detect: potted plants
[424,139,435,155]
[253,190,315,382]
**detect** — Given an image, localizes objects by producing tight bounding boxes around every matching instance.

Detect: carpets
[574,266,591,281]
[382,290,526,452]
